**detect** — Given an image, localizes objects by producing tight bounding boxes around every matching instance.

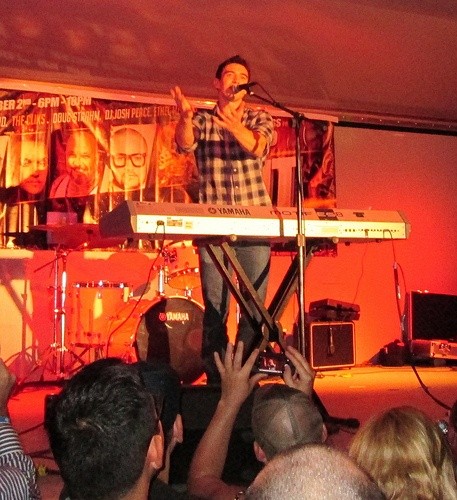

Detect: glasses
[111,153,146,167]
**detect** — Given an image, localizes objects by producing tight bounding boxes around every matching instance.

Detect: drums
[161,241,202,291]
[66,282,134,348]
[103,294,213,380]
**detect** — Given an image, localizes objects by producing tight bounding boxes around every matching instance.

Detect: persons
[187,341,457,500]
[43,355,165,500]
[8,125,49,196]
[105,129,149,189]
[62,132,100,192]
[0,356,39,500]
[168,56,276,397]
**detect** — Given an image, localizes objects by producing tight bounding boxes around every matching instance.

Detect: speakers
[294,322,356,370]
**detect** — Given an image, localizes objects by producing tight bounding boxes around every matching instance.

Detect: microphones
[328,328,335,355]
[233,82,257,93]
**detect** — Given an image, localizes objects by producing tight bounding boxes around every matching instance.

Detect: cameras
[255,352,291,376]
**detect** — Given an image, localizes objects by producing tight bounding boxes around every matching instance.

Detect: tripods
[5,235,90,389]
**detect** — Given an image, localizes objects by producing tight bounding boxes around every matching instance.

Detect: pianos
[98,200,411,249]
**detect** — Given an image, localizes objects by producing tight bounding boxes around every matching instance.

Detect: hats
[250,382,322,461]
